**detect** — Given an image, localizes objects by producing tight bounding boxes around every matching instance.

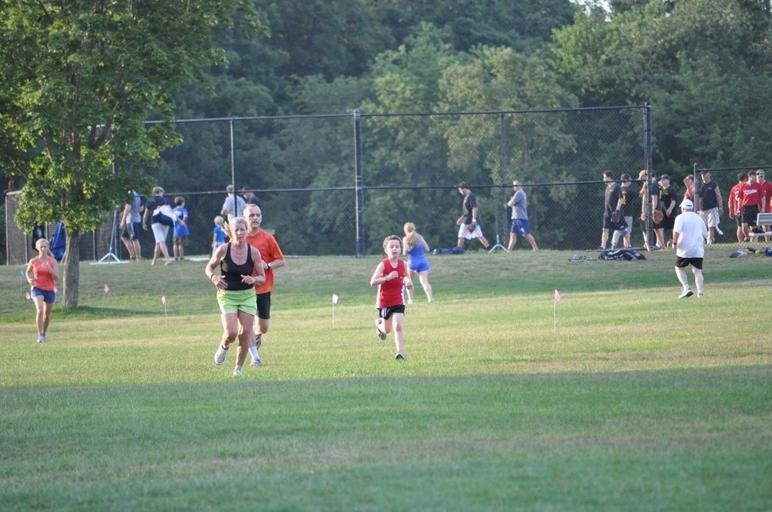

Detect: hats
[678,199,694,210]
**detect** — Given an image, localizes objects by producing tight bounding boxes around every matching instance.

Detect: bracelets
[208,272,214,283]
[672,241,677,245]
[267,262,272,270]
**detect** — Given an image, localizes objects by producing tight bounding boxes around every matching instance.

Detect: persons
[671,200,709,300]
[403,221,436,302]
[204,217,266,378]
[118,180,263,260]
[26,238,60,341]
[368,235,415,361]
[454,181,493,251]
[599,169,772,251]
[242,203,286,366]
[506,180,538,251]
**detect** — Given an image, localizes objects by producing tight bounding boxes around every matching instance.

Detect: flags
[332,293,339,303]
[19,270,167,305]
[554,288,559,301]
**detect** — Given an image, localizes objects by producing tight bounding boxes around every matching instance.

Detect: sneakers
[679,288,693,299]
[214,332,261,367]
[377,318,386,340]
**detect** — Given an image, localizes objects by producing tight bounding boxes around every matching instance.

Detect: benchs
[749,213,772,243]
